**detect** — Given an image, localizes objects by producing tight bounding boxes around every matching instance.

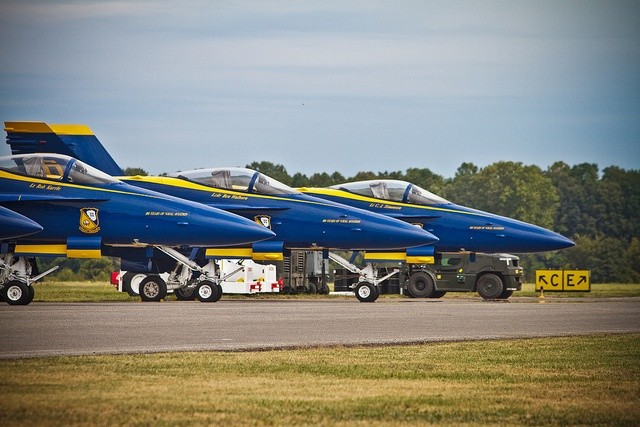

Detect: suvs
[403,251,523,300]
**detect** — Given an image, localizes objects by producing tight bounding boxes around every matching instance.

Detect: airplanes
[0,119,439,303]
[1,153,279,306]
[292,179,575,302]
